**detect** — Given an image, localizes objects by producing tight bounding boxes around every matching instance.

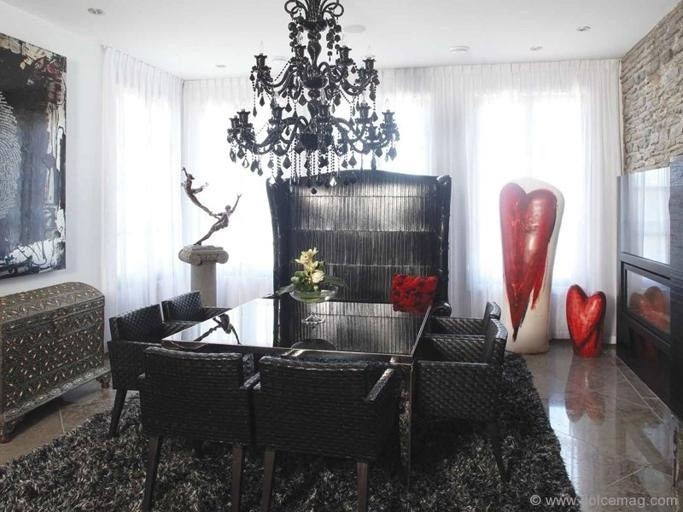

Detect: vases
[285,288,338,326]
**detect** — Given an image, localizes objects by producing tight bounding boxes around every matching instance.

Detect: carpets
[0,346,580,512]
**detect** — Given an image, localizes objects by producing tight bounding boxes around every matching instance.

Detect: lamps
[224,0,399,196]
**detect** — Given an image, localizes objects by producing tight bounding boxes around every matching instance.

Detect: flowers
[269,246,353,295]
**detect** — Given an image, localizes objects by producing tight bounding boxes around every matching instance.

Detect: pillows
[391,272,438,311]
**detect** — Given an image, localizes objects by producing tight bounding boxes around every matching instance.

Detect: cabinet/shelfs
[0,281,105,419]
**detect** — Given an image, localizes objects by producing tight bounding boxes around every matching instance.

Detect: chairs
[265,169,453,316]
[103,292,510,512]
